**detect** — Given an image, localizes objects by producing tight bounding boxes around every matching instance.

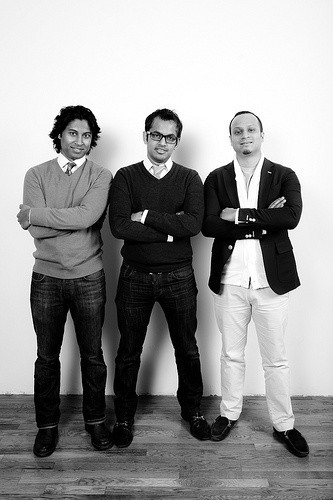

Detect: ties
[65,162,75,175]
[153,166,166,179]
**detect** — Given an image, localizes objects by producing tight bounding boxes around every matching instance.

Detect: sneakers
[272,427,309,458]
[84,417,114,450]
[181,412,211,440]
[33,425,59,457]
[210,415,237,442]
[111,417,135,448]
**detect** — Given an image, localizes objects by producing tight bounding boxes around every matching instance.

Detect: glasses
[146,131,178,144]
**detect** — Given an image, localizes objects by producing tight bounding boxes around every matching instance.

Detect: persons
[16,106,113,458]
[202,110,310,458]
[106,109,211,447]
